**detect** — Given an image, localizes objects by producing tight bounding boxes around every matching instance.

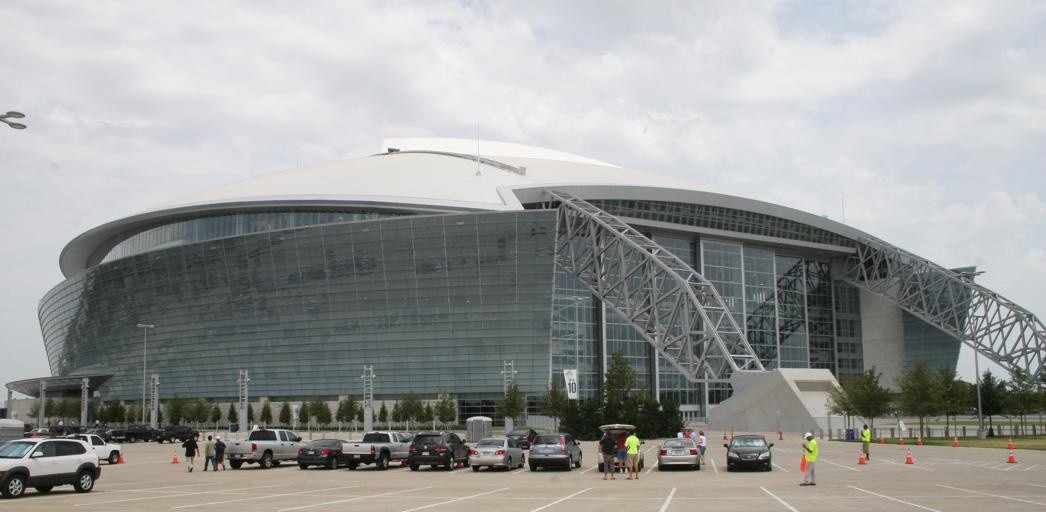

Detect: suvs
[63,433,123,464]
[408,432,471,471]
[0,438,101,497]
[597,423,645,473]
[529,432,583,470]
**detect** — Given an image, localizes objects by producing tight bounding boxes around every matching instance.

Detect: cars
[467,437,525,471]
[23,423,111,438]
[507,427,538,446]
[297,438,360,469]
[723,434,774,471]
[655,437,700,471]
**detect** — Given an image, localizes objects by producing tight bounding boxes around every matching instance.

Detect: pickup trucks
[112,423,157,443]
[152,425,199,443]
[341,430,416,469]
[223,428,311,468]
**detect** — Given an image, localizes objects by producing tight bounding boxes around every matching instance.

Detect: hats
[804,432,812,439]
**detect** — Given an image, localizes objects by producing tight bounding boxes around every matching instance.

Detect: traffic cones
[879,436,885,444]
[858,447,867,464]
[898,438,905,444]
[1005,447,1018,463]
[916,433,924,445]
[952,434,960,447]
[172,450,179,463]
[905,447,914,464]
[1007,435,1016,448]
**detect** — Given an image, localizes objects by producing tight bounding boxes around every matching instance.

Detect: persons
[530,431,538,442]
[182,435,199,472]
[861,425,870,460]
[203,436,216,471]
[600,432,617,480]
[799,432,817,486]
[678,429,706,465]
[624,431,640,480]
[214,436,226,470]
[616,432,628,474]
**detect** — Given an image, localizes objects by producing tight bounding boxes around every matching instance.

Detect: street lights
[235,368,251,431]
[135,323,154,425]
[37,381,47,428]
[0,110,27,129]
[958,269,988,440]
[360,365,377,431]
[5,388,13,419]
[78,377,90,429]
[148,372,160,426]
[500,360,519,400]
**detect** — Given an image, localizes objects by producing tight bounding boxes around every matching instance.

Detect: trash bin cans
[846,429,854,440]
